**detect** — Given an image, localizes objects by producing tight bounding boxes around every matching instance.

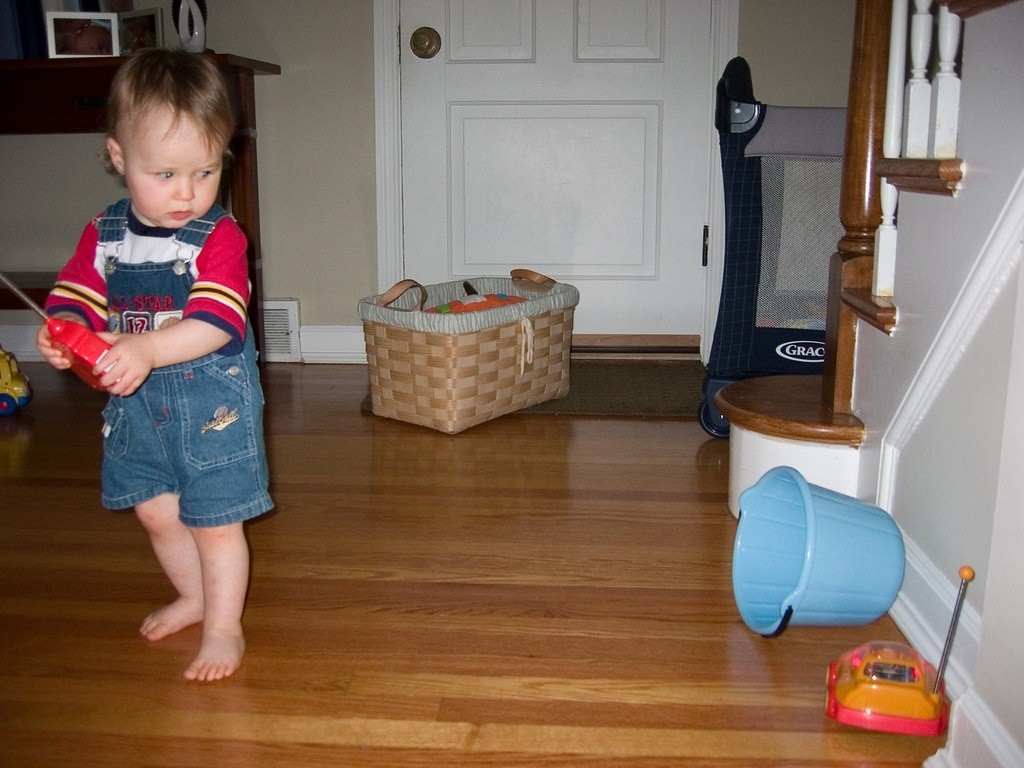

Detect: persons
[37,49,275,681]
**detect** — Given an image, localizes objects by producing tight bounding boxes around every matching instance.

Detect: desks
[0,53,282,371]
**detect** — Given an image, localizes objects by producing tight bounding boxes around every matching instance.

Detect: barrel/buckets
[731,465,907,638]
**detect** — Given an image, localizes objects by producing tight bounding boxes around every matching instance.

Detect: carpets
[360,360,707,417]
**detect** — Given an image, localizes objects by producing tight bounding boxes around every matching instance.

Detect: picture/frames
[119,7,164,57]
[45,11,121,59]
